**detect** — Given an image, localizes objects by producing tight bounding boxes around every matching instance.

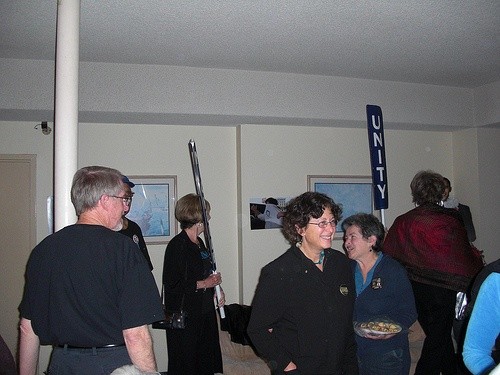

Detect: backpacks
[453,258,500,363]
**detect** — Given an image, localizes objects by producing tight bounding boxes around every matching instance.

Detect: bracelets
[204,280,206,291]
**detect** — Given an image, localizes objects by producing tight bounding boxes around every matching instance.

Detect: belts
[57,343,126,349]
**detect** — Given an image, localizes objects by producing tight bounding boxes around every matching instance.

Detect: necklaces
[315,251,325,264]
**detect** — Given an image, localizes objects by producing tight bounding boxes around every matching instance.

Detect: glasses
[307,220,336,228]
[100,194,131,206]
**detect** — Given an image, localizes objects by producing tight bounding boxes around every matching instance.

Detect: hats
[120,175,135,188]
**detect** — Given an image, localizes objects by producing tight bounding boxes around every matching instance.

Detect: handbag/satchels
[151,312,185,329]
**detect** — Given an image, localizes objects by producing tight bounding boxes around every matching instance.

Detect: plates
[359,322,402,336]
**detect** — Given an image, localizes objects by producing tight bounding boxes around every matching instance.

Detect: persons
[382,171,481,375]
[441,178,476,242]
[453,258,500,375]
[19,166,166,375]
[162,193,226,375]
[342,214,426,375]
[248,192,359,375]
[118,175,154,271]
[252,197,284,229]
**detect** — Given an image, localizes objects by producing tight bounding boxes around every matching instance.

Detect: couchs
[217,309,457,375]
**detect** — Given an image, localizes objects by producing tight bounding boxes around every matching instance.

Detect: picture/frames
[126,175,178,245]
[307,175,378,240]
